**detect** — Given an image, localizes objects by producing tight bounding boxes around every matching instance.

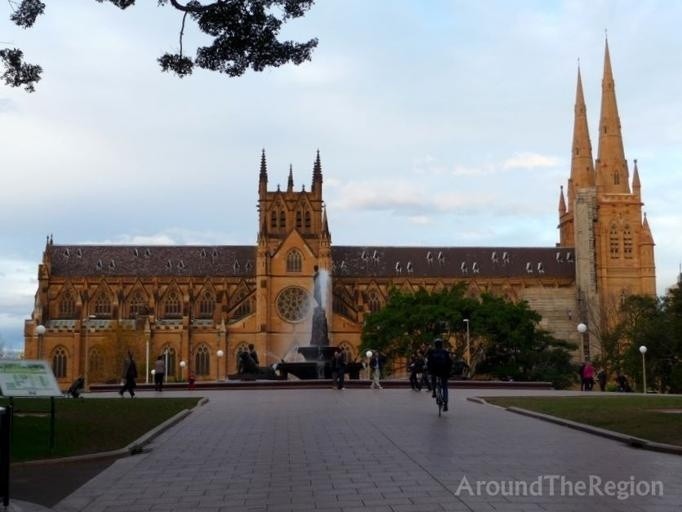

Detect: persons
[579,359,635,393]
[406,338,454,412]
[188,371,196,390]
[330,346,385,392]
[119,353,139,398]
[155,355,165,392]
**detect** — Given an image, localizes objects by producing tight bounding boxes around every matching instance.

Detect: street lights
[216,350,224,380]
[640,346,648,394]
[367,350,373,380]
[180,361,185,381]
[36,324,46,361]
[151,369,155,383]
[463,319,474,367]
[578,322,588,364]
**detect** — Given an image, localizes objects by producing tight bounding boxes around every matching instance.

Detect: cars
[407,352,471,379]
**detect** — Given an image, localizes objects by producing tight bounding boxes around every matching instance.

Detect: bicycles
[424,364,448,416]
